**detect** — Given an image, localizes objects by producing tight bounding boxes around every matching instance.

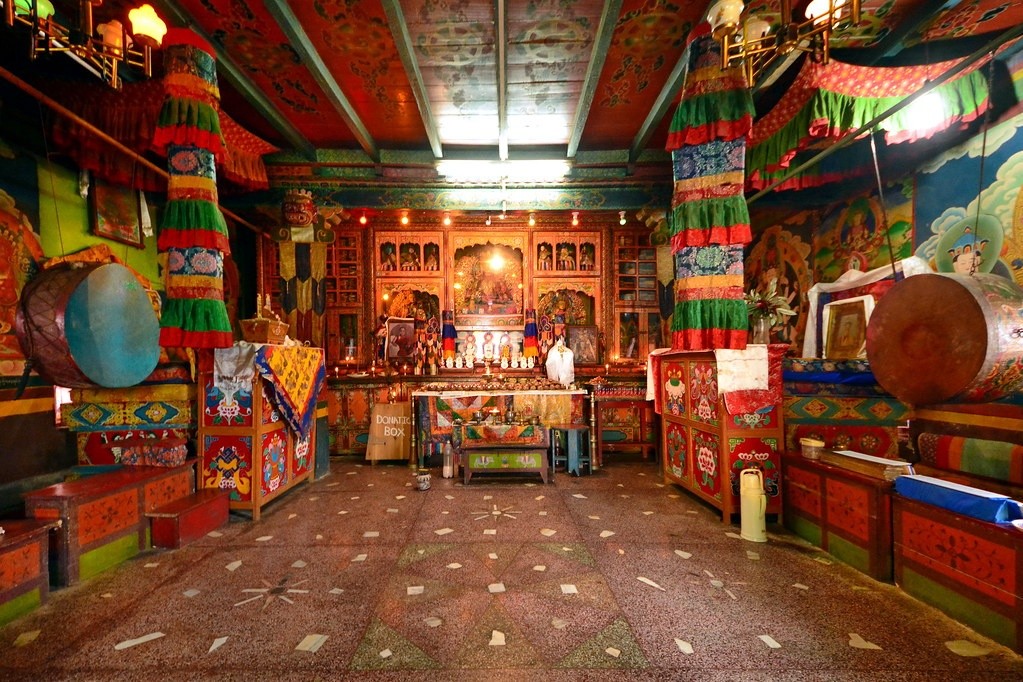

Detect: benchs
[145,487,235,548]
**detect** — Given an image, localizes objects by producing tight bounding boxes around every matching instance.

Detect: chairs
[450,423,551,485]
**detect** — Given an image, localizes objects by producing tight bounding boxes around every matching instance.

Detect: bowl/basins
[800,438,825,459]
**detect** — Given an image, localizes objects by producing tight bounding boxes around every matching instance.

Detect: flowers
[744,280,796,322]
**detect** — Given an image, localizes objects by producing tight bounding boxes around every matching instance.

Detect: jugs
[740,469,767,543]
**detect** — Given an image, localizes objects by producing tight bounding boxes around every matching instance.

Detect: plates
[346,373,369,378]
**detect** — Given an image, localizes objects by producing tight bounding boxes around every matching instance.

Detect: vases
[751,315,772,343]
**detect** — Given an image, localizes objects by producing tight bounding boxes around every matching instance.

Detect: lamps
[4,0,168,90]
[706,1,863,91]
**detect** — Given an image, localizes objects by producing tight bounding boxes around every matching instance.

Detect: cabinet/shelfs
[611,210,664,374]
[652,344,791,526]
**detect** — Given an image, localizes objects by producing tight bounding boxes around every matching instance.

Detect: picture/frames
[88,175,145,249]
[825,298,867,361]
[567,325,599,366]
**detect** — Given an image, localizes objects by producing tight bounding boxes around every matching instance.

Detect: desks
[779,450,1023,659]
[197,344,324,523]
[324,368,656,459]
[410,384,600,476]
[17,458,203,585]
[0,518,63,624]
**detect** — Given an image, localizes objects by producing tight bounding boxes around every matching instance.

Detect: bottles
[443,441,454,479]
